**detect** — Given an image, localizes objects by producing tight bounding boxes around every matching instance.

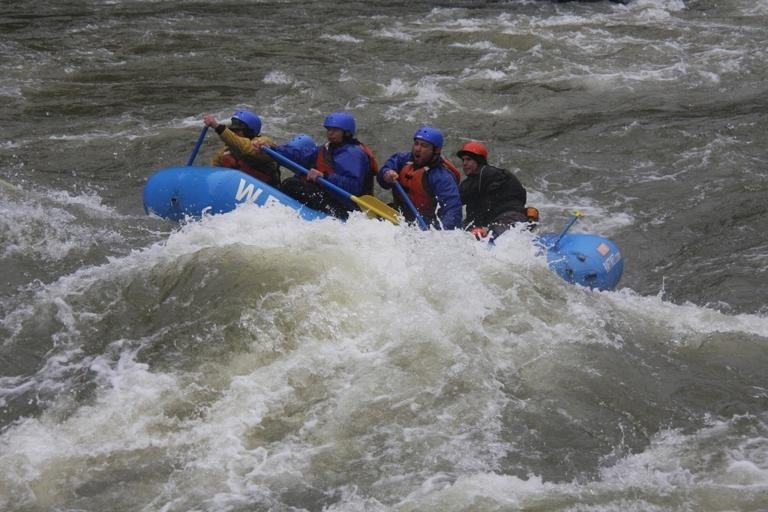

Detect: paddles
[259,144,402,226]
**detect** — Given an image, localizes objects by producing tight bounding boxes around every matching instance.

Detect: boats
[144,166,624,303]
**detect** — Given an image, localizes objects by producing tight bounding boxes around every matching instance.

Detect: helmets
[414,127,443,147]
[324,113,356,134]
[456,142,488,161]
[231,110,261,136]
[287,133,316,156]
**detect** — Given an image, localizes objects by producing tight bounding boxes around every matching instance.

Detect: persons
[203,110,281,190]
[253,112,378,223]
[457,142,538,246]
[286,134,317,179]
[377,128,462,230]
